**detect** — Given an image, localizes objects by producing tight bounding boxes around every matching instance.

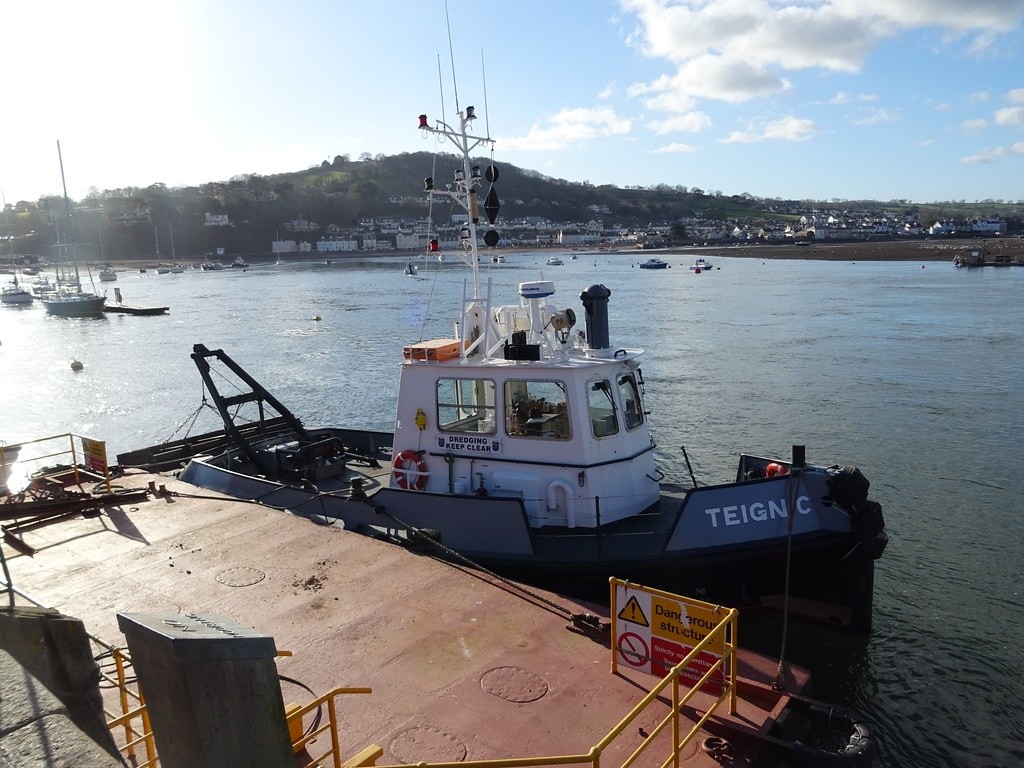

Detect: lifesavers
[393,449,427,491]
[765,463,786,479]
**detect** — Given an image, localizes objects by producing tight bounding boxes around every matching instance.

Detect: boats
[688,258,713,270]
[106,302,170,316]
[545,257,562,265]
[175,0,888,669]
[491,255,505,263]
[139,269,146,273]
[570,255,577,259]
[953,248,1019,267]
[403,266,417,275]
[438,254,447,261]
[99,268,117,281]
[795,241,812,246]
[639,258,668,268]
[232,257,250,268]
[22,268,60,295]
[201,261,225,271]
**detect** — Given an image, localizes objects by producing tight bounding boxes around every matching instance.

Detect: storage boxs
[401,338,471,362]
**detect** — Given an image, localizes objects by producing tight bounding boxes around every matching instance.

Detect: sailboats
[155,226,170,274]
[170,221,183,273]
[38,141,108,316]
[94,224,108,270]
[277,231,287,265]
[0,193,33,304]
